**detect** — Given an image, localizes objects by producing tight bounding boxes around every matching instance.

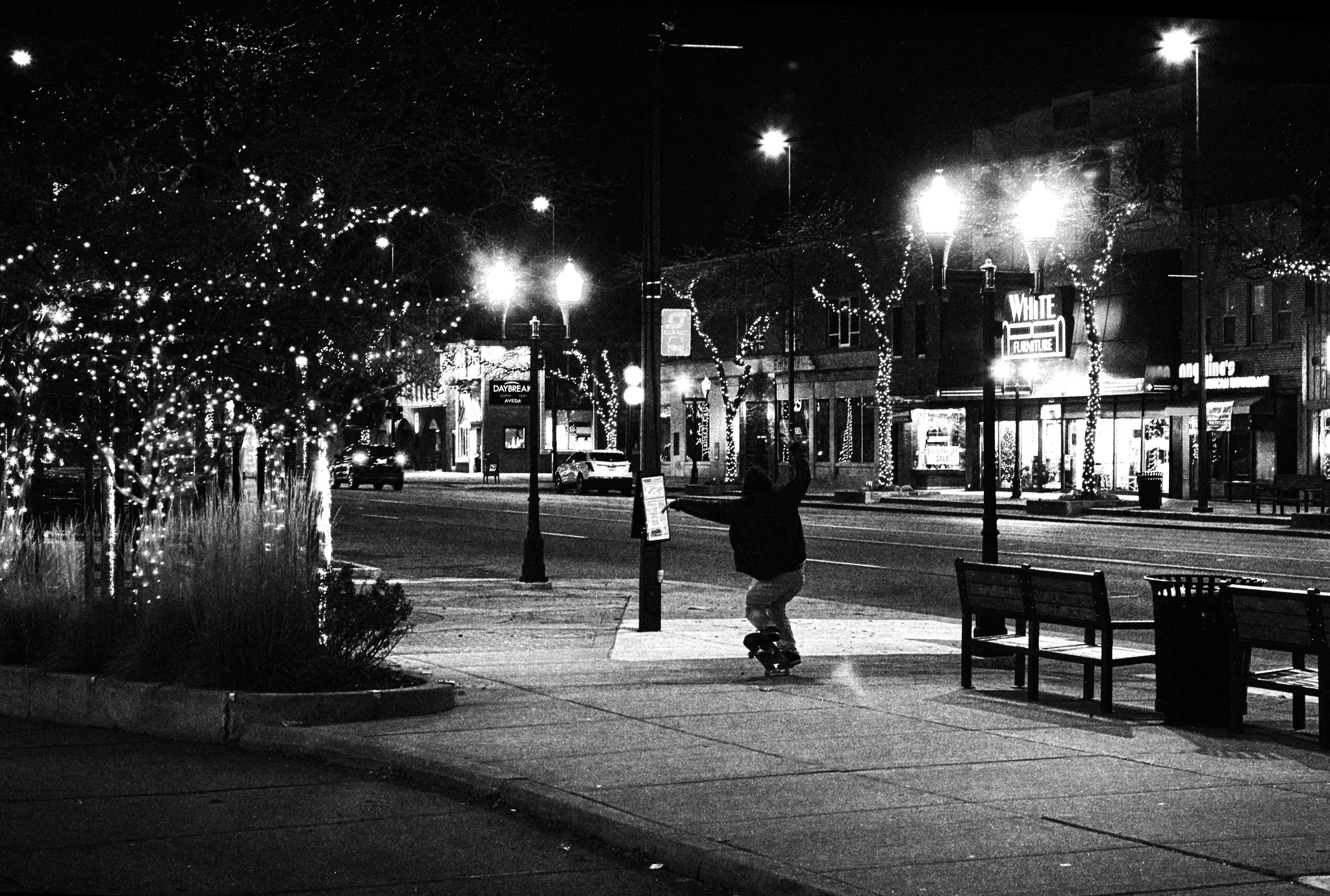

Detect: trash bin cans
[1144,571,1269,727]
[1135,470,1164,510]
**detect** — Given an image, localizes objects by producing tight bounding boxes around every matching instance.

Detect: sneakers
[777,653,801,670]
[755,625,780,642]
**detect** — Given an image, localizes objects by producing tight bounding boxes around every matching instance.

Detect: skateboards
[742,631,791,678]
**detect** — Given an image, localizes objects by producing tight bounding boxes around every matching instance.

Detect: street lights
[676,370,710,484]
[756,123,804,468]
[617,344,645,462]
[467,246,590,594]
[992,358,1037,504]
[1162,22,1220,517]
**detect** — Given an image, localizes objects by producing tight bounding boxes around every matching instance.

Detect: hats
[743,466,773,494]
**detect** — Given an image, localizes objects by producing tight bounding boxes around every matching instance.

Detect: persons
[660,441,812,665]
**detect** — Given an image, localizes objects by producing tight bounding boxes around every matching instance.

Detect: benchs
[1254,473,1326,515]
[951,556,1156,716]
[1218,575,1330,751]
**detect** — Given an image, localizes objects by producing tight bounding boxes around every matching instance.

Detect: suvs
[328,442,408,492]
[555,446,633,495]
